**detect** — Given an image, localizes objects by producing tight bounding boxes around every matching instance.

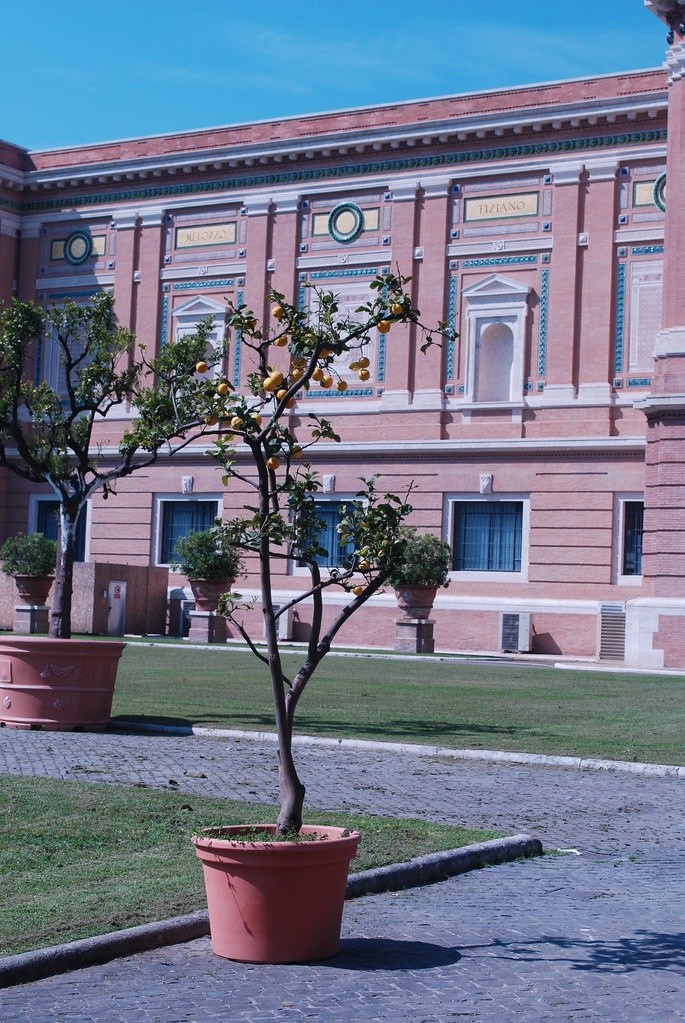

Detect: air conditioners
[498,612,533,651]
[262,603,294,639]
[179,600,197,636]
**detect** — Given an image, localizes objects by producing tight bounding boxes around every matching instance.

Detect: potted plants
[0,289,232,737]
[0,531,57,605]
[172,260,462,965]
[171,526,244,611]
[377,525,454,607]
